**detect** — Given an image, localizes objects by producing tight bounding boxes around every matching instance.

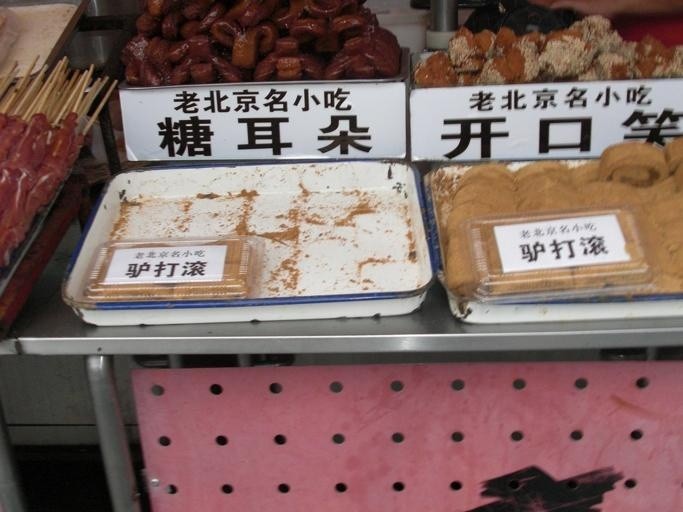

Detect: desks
[0,218,82,512]
[15,217,681,512]
